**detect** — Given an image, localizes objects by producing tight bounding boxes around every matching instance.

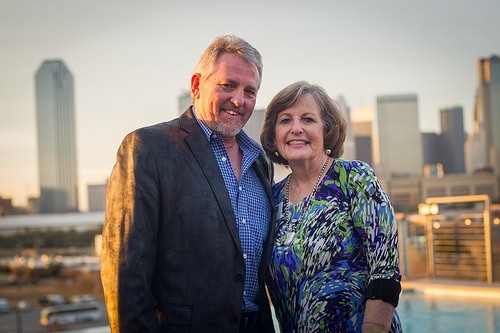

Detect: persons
[259,82,403,333]
[100,34,274,333]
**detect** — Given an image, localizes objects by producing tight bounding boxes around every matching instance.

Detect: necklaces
[284,156,329,228]
